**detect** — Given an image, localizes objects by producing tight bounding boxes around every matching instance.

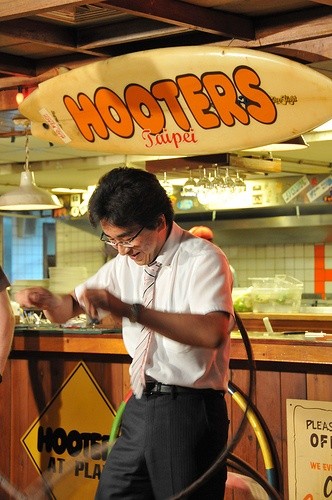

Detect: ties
[129,263,161,399]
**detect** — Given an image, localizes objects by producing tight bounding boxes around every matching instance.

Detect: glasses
[100,225,146,248]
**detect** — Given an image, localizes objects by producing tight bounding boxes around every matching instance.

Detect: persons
[0,265,16,385]
[13,166,236,500]
[186,226,236,293]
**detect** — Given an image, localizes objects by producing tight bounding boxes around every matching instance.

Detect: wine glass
[160,168,246,196]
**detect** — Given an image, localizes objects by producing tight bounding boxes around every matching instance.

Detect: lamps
[0,124,63,210]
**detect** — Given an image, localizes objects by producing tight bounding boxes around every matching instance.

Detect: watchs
[130,303,146,323]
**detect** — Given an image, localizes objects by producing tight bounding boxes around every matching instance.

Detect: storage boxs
[230,275,303,314]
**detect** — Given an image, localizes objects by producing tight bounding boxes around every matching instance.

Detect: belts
[144,381,225,397]
